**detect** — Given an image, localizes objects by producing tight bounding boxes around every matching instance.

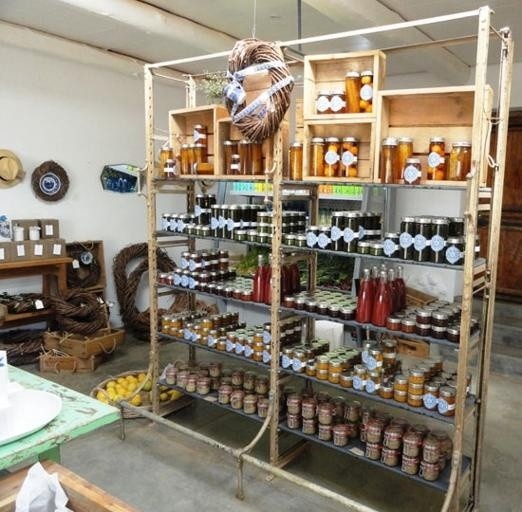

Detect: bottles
[249,249,300,305]
[353,265,406,327]
[286,70,472,184]
[157,123,271,178]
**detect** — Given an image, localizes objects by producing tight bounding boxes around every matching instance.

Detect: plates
[38,172,62,196]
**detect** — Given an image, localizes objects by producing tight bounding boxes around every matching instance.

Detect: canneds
[380,137,413,183]
[310,137,359,177]
[316,71,373,113]
[162,303,472,416]
[403,158,422,185]
[289,143,302,180]
[162,179,466,265]
[427,137,471,181]
[165,362,453,481]
[157,124,214,175]
[158,246,479,343]
[223,138,264,175]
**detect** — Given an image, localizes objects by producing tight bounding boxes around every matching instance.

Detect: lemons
[95,372,183,406]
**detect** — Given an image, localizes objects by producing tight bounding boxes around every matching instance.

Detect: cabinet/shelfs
[0,257,73,330]
